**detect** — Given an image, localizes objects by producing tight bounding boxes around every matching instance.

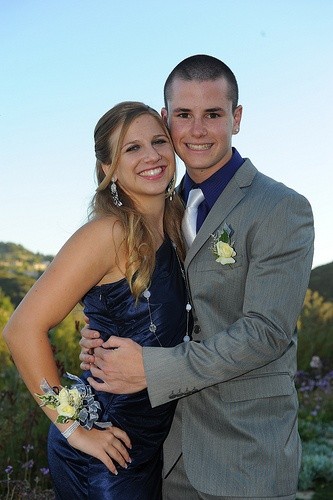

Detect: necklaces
[143,237,191,349]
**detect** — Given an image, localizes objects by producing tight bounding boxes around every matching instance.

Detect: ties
[178,186,207,249]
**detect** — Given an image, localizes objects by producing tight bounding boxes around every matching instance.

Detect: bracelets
[64,421,79,438]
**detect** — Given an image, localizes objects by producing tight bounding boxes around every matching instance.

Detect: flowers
[34,371,112,431]
[209,222,237,269]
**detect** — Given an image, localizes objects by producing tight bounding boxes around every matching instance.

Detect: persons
[79,55,314,500]
[2,102,194,500]
